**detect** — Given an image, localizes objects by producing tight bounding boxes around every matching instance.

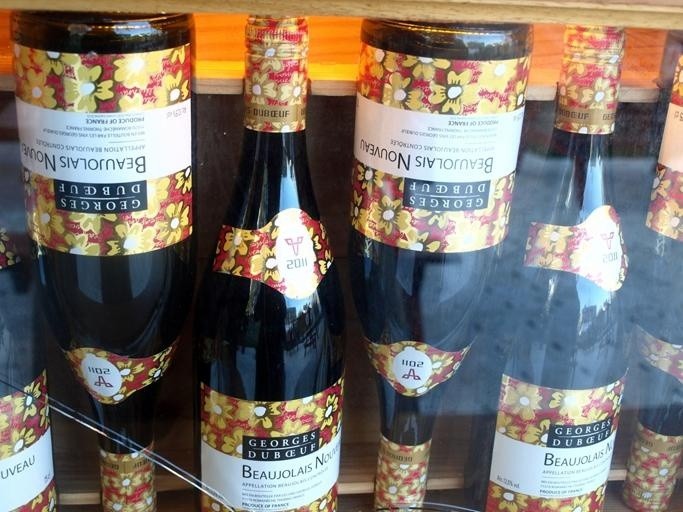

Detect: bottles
[0,225,58,510]
[10,12,198,511]
[621,29,683,510]
[351,17,534,512]
[465,25,636,511]
[193,14,346,512]
